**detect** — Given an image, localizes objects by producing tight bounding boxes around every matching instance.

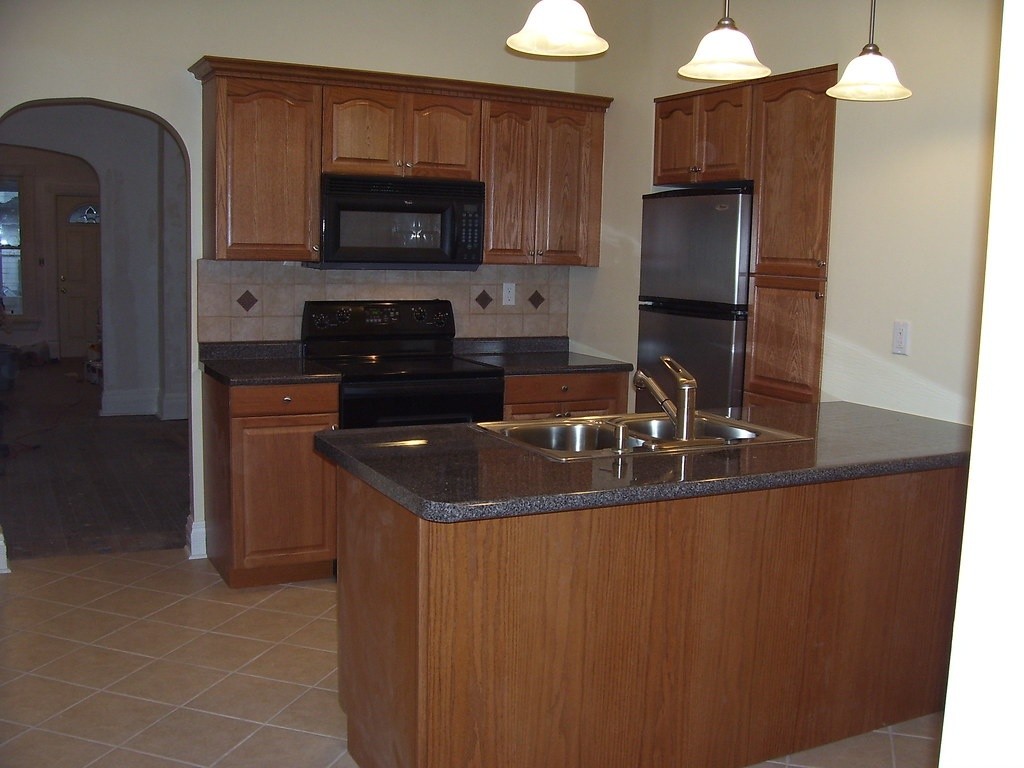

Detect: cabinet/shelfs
[741,65,839,407]
[202,375,339,570]
[202,77,323,262]
[323,85,482,182]
[654,84,752,187]
[504,371,629,419]
[480,100,592,267]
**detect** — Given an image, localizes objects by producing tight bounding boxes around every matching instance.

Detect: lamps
[825,0,910,102]
[677,0,771,80]
[505,0,609,58]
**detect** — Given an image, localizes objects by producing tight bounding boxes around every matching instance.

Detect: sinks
[503,422,646,452]
[627,416,760,438]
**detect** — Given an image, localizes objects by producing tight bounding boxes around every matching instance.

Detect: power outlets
[502,283,516,306]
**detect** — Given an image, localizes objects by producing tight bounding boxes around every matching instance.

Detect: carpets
[0,357,190,560]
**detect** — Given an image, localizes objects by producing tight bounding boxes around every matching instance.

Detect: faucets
[632,355,698,442]
